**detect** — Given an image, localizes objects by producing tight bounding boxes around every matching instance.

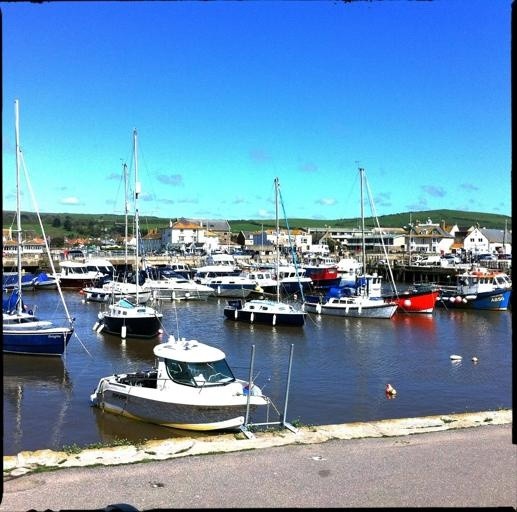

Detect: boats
[90,339,270,431]
